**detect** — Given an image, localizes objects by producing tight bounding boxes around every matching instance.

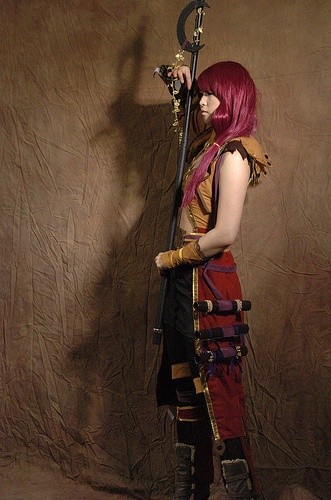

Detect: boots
[172,441,211,499]
[217,457,256,500]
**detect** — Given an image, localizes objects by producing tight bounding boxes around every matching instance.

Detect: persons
[154,60,271,500]
